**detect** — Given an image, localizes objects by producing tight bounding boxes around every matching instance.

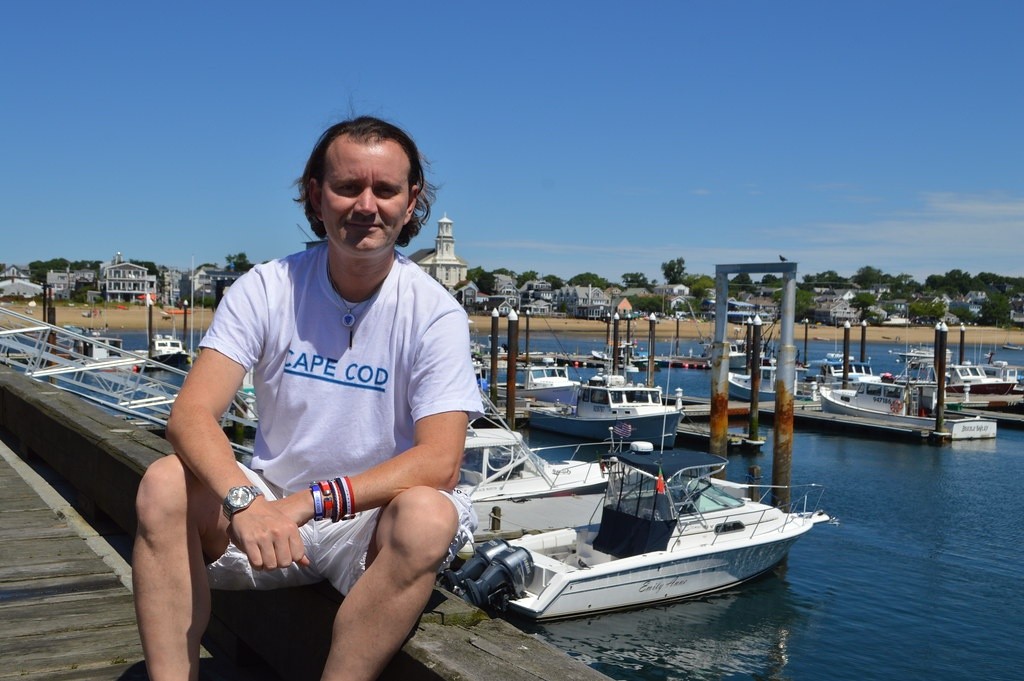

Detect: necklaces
[327,259,389,349]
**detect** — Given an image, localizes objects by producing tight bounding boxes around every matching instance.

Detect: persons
[131,112,487,681]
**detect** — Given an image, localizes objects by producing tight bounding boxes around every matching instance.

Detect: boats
[489,308,686,449]
[67,296,192,369]
[431,399,832,622]
[726,312,1023,442]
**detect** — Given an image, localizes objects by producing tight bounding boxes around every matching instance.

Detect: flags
[614,421,632,437]
[656,467,665,494]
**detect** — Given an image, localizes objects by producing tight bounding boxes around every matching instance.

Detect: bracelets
[310,475,357,521]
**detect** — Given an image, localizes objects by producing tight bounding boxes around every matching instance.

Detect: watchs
[223,486,265,520]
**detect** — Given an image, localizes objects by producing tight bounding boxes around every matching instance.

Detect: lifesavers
[890,400,903,414]
